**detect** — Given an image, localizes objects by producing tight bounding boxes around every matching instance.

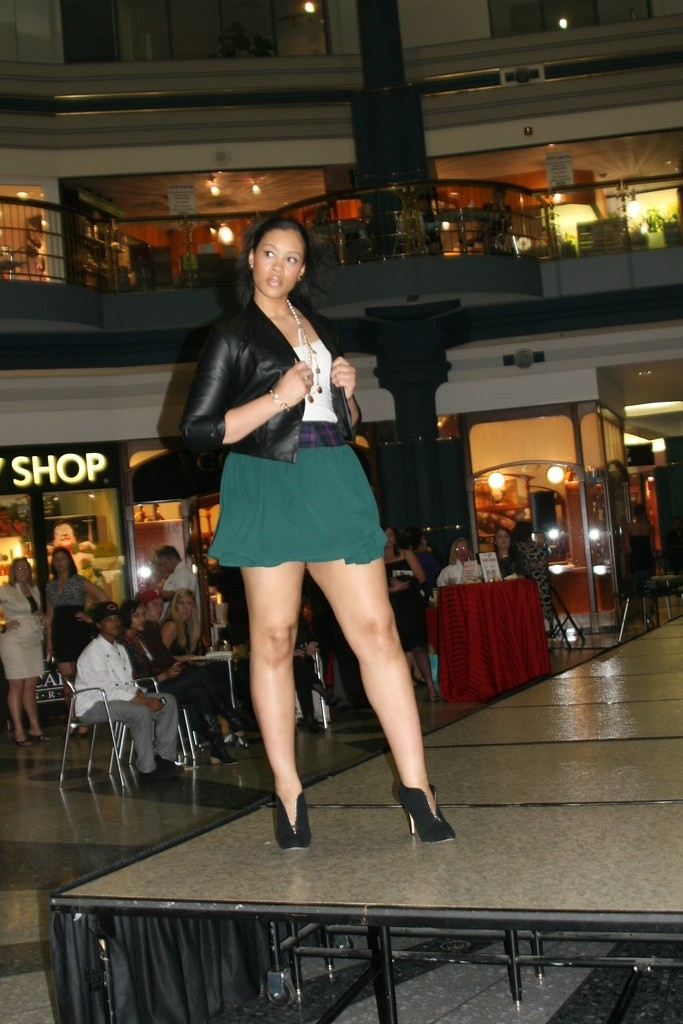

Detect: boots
[210,689,250,732]
[203,712,239,766]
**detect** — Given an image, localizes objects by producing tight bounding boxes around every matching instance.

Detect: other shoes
[428,689,440,702]
[140,769,170,784]
[642,616,655,630]
[304,718,322,734]
[155,756,184,774]
[77,726,92,737]
[351,692,371,709]
[412,675,426,689]
[63,723,77,738]
[325,695,349,710]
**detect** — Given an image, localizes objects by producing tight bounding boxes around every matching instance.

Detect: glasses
[455,547,466,551]
[16,567,29,570]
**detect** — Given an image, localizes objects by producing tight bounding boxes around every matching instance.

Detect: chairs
[58,675,199,786]
[650,544,683,622]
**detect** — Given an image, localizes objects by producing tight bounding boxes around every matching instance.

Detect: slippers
[28,731,51,741]
[12,737,34,746]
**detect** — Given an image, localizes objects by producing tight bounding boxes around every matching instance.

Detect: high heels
[397,781,454,843]
[274,791,311,849]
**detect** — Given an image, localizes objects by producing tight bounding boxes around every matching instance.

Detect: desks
[316,219,368,265]
[436,577,551,702]
[435,207,488,255]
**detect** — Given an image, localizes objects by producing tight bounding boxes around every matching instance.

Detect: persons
[662,516,683,575]
[416,194,440,255]
[120,545,249,766]
[0,557,54,746]
[475,187,517,255]
[512,521,555,621]
[379,522,446,703]
[625,502,660,626]
[73,600,185,788]
[492,526,533,579]
[310,203,401,267]
[176,217,455,853]
[291,612,345,737]
[43,546,110,738]
[436,537,484,586]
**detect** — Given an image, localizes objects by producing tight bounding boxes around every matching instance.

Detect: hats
[140,589,162,601]
[94,601,121,622]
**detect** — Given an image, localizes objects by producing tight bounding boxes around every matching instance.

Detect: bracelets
[1,625,6,633]
[268,389,291,412]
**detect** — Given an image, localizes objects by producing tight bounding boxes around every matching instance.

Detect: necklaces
[287,299,323,403]
